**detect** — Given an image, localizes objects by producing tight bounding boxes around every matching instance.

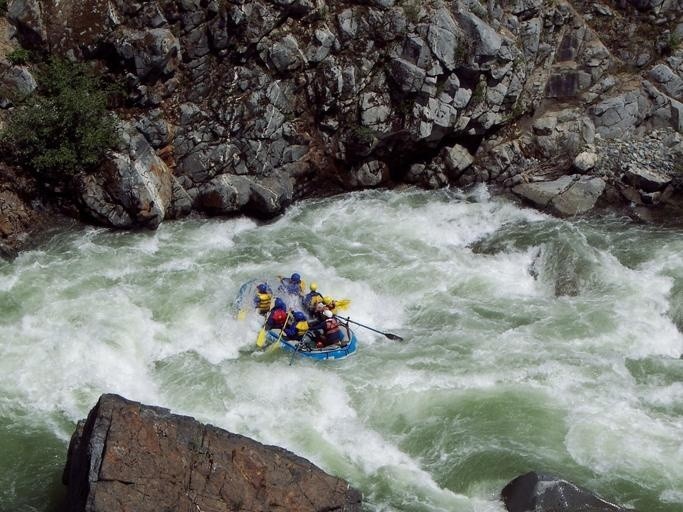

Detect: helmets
[323,310,332,317]
[310,283,317,291]
[259,284,267,292]
[293,312,304,318]
[275,299,283,308]
[291,274,300,282]
[324,297,333,306]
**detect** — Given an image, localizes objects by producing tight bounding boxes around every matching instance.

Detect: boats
[234,275,359,362]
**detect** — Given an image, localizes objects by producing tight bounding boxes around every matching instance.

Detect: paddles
[239,280,351,367]
[337,315,403,341]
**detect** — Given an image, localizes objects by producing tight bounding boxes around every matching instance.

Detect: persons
[253,273,344,348]
